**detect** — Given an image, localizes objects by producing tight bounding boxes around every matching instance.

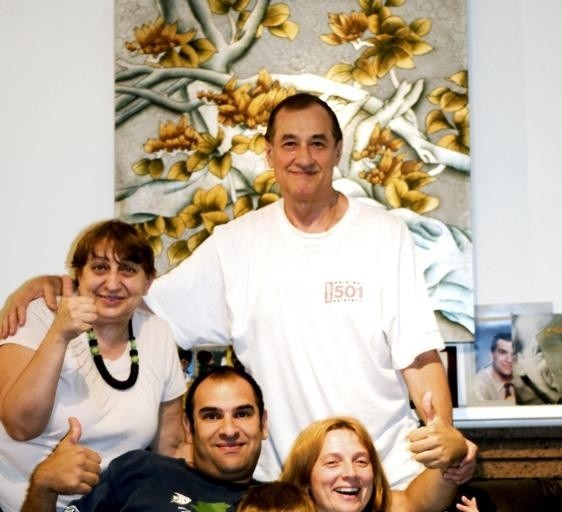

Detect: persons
[452,494,480,512]
[0,91,479,492]
[236,479,318,511]
[278,417,391,512]
[1,217,188,512]
[18,366,467,512]
[178,350,216,377]
[472,333,515,402]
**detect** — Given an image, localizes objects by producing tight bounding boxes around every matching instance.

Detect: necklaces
[85,317,140,389]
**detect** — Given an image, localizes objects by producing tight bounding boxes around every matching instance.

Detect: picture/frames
[456,302,555,409]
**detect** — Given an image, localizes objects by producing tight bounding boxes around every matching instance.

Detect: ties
[504,382,511,399]
